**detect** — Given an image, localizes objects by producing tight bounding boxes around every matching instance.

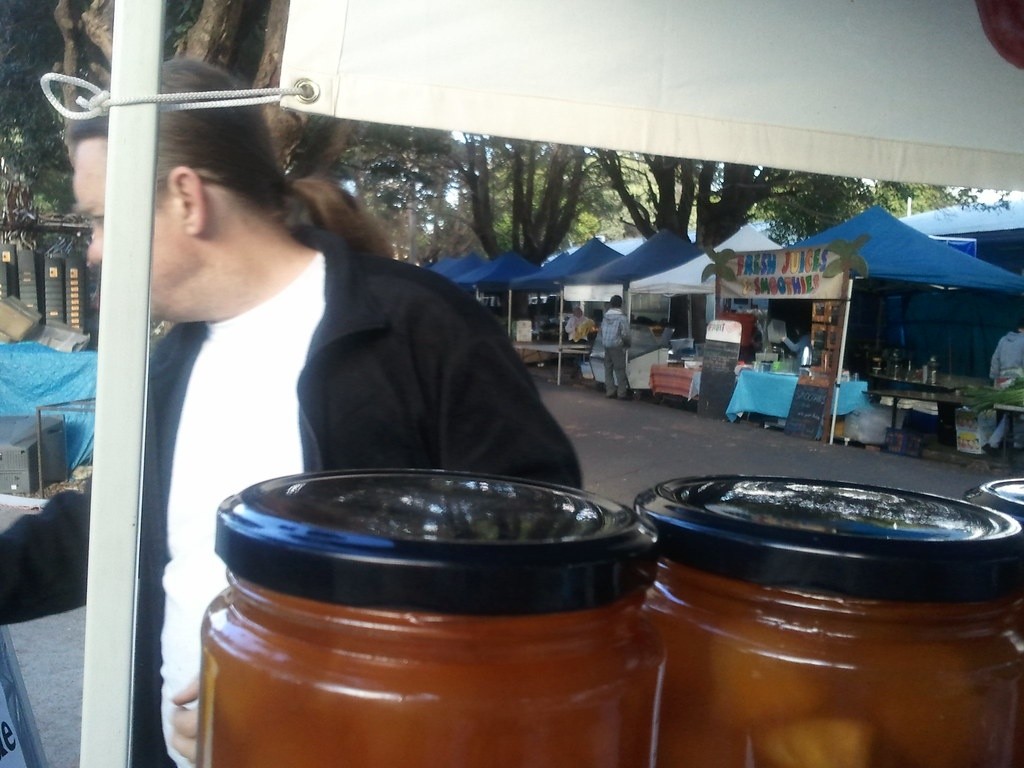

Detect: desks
[512,340,1024,469]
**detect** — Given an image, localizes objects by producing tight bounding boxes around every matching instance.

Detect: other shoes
[982,443,1002,458]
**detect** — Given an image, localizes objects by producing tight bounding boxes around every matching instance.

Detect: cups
[853,372,859,381]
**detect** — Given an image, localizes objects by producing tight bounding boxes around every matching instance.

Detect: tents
[506,237,626,339]
[623,223,784,371]
[420,252,487,281]
[454,251,543,302]
[557,229,706,387]
[784,204,1024,445]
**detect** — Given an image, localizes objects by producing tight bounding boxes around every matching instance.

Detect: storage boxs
[0,292,43,344]
[955,407,996,430]
[754,351,778,361]
[883,427,922,457]
[670,338,695,351]
[767,319,786,344]
[957,430,995,454]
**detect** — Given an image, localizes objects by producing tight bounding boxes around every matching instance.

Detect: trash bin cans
[0,414,71,495]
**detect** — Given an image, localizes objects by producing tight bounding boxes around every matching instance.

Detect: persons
[0,59,582,768]
[564,307,595,378]
[602,295,632,399]
[981,318,1024,456]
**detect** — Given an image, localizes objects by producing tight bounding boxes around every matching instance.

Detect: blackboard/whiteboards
[696,340,740,420]
[783,383,830,440]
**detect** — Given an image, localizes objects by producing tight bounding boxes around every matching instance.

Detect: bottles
[927,358,936,384]
